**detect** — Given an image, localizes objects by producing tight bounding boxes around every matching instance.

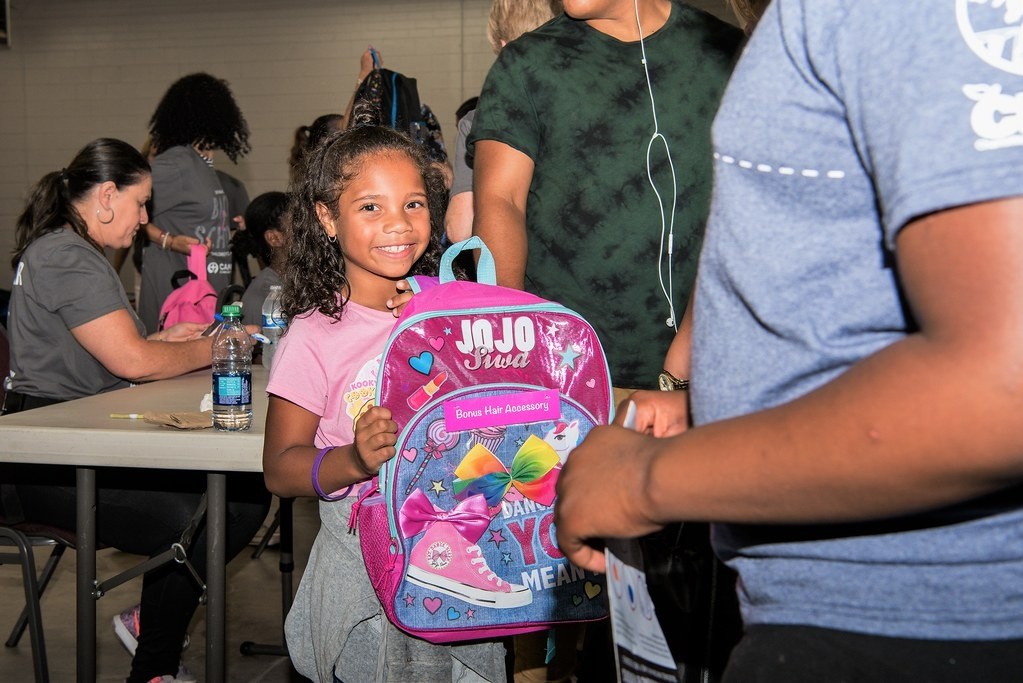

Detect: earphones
[665,308,675,327]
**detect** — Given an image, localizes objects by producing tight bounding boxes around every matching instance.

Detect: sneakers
[112,602,195,683]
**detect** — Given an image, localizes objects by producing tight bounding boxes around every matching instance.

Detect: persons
[554,0,1023,683]
[197,142,251,285]
[112,138,153,272]
[7,139,260,682]
[139,71,253,332]
[263,124,509,683]
[432,0,564,244]
[474,0,749,683]
[234,44,385,544]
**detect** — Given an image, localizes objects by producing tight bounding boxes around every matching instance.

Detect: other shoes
[250,525,282,546]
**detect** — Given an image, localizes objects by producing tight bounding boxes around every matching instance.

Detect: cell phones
[200,301,243,338]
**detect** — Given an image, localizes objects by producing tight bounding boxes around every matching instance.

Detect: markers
[214,314,274,345]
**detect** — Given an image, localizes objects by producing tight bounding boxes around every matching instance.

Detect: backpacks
[158,243,219,333]
[348,239,612,644]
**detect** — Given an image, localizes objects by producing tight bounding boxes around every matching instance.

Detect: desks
[0,365,301,683]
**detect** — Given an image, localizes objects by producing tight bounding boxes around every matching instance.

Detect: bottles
[262,285,289,371]
[211,305,254,432]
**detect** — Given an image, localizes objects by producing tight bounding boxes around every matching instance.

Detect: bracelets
[312,446,355,501]
[159,332,162,342]
[157,229,176,250]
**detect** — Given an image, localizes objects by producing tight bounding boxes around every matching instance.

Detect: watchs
[658,370,690,391]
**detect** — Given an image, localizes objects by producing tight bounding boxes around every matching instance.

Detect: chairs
[0,287,110,683]
